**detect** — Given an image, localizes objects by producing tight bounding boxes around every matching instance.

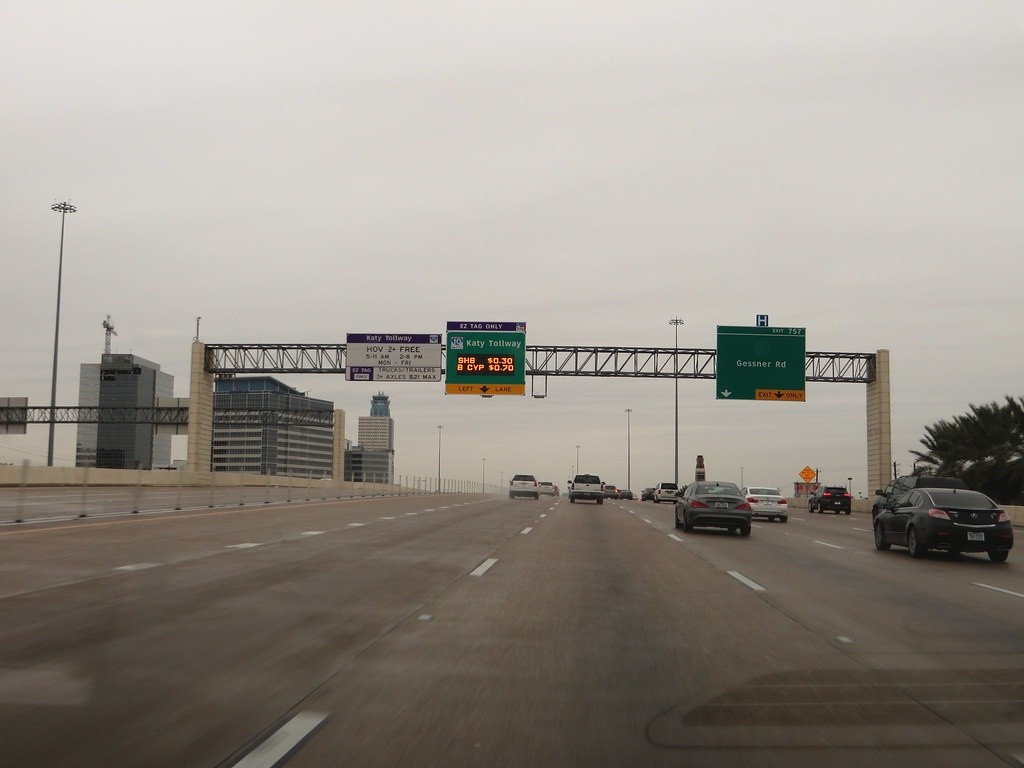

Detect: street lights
[437,425,444,493]
[666,315,686,484]
[48,197,78,465]
[482,458,486,493]
[575,445,581,474]
[625,408,633,490]
[501,471,503,494]
[571,465,574,480]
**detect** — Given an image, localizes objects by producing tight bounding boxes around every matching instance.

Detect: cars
[736,486,790,523]
[874,488,1014,563]
[568,473,606,504]
[538,481,561,497]
[674,480,752,537]
[508,474,541,500]
[603,485,618,500]
[641,488,656,501]
[618,489,633,500]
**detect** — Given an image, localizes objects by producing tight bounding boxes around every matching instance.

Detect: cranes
[102,311,118,354]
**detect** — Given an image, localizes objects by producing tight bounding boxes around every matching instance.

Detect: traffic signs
[444,322,526,395]
[716,324,806,403]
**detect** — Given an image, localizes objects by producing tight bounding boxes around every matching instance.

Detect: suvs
[808,485,852,514]
[654,482,679,504]
[872,472,971,522]
